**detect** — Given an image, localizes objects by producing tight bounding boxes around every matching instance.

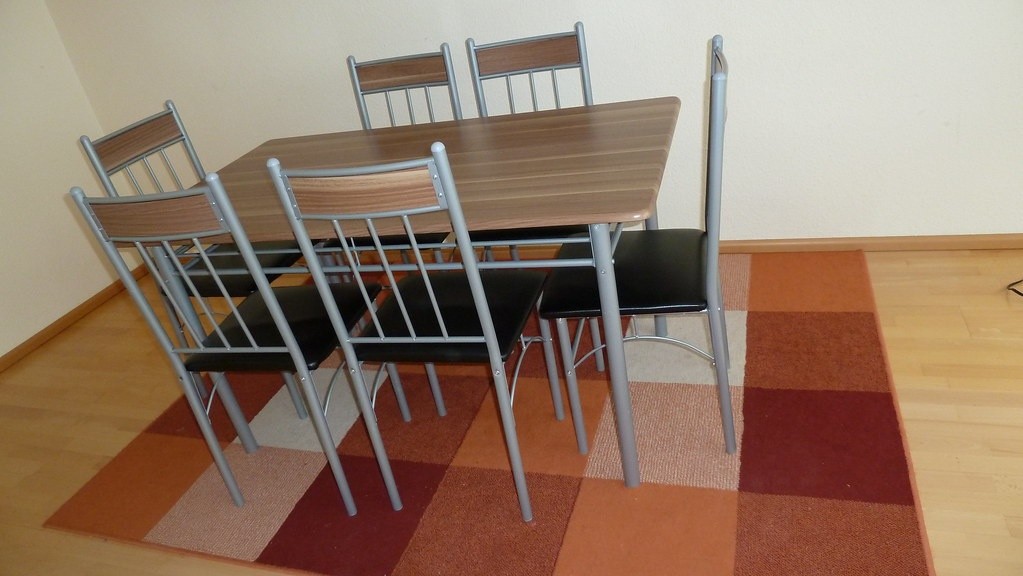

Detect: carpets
[40,249,937,576]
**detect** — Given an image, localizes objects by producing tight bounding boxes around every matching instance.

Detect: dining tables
[151,96,682,490]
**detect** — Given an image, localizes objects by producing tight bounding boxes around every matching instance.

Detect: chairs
[69,171,413,516]
[79,100,329,401]
[538,34,739,489]
[264,140,568,522]
[464,21,594,268]
[314,42,464,285]
